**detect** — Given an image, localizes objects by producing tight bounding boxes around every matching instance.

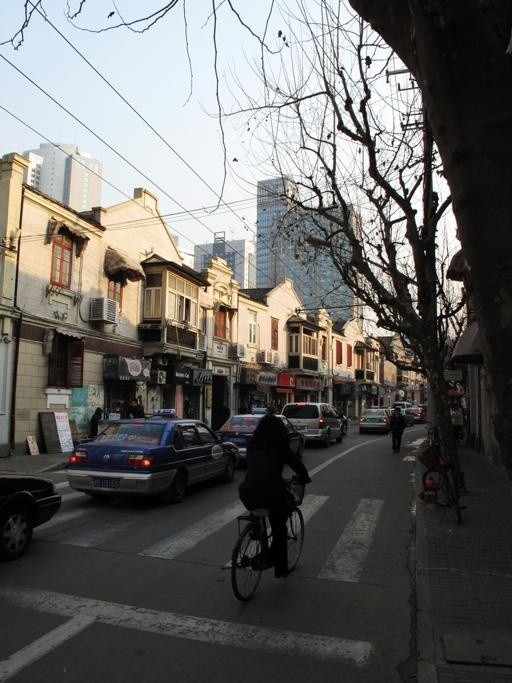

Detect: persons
[212,397,230,430]
[128,398,145,418]
[238,412,311,578]
[90,407,104,437]
[389,406,406,454]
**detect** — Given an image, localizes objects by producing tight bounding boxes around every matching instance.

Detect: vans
[280,402,342,447]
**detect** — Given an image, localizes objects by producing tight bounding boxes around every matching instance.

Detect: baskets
[283,478,305,506]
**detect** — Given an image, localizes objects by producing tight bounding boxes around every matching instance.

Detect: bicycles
[220,475,311,603]
[419,436,465,523]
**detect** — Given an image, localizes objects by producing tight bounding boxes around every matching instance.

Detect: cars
[391,401,428,425]
[214,408,304,465]
[332,405,348,435]
[0,468,62,558]
[66,408,241,503]
[359,408,391,434]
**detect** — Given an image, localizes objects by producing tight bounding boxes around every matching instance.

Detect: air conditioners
[89,296,120,324]
[228,343,246,358]
[151,369,168,385]
[258,350,273,363]
[272,350,281,365]
